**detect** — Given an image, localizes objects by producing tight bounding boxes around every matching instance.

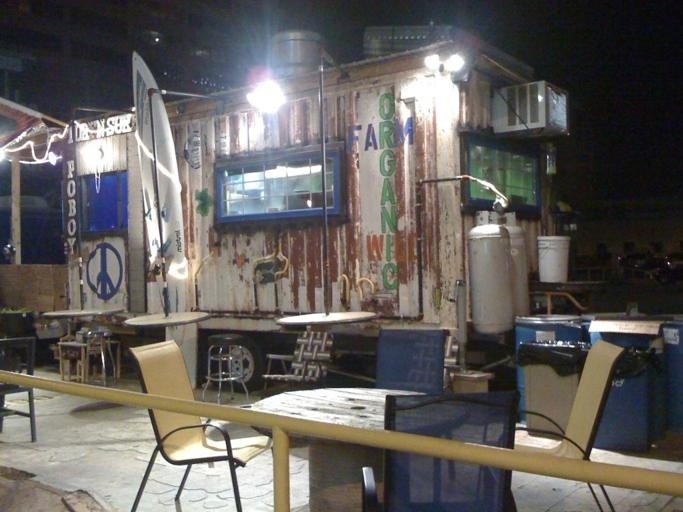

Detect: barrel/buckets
[536,235,572,284]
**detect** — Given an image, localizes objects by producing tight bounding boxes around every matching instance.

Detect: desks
[57,341,121,382]
[248,388,470,512]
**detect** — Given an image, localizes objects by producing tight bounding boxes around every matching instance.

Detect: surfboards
[132,50,189,346]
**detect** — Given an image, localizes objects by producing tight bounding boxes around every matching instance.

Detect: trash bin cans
[513,340,651,450]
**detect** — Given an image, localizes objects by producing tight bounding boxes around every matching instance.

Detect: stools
[202,334,250,405]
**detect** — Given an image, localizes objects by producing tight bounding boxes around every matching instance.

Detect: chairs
[77,331,116,385]
[374,327,452,388]
[261,329,334,398]
[0,336,36,442]
[515,339,626,511]
[127,340,273,512]
[382,387,522,512]
